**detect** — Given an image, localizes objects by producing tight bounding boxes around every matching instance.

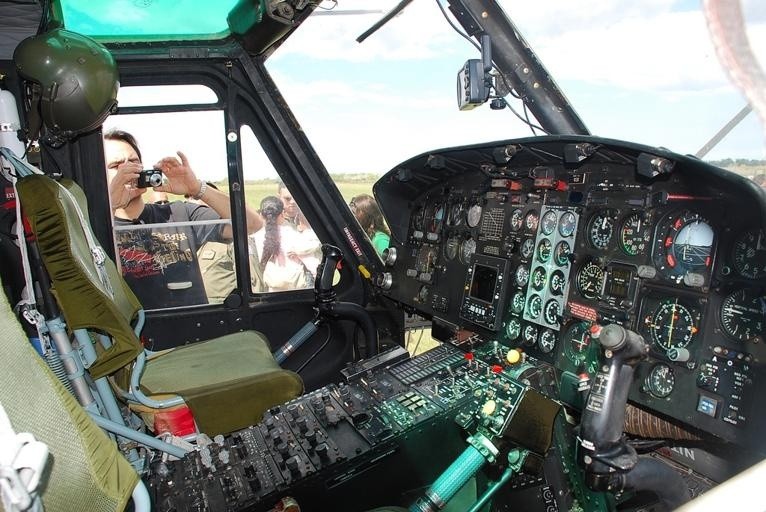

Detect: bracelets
[192,175,208,200]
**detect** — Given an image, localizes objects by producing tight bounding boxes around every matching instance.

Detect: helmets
[13,29,121,148]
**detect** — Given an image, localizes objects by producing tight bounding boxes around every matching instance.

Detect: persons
[182,181,268,304]
[251,181,393,294]
[151,191,170,207]
[98,127,267,313]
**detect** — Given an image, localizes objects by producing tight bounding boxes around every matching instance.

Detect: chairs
[16,175,305,442]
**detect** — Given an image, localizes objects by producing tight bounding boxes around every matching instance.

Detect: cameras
[137,169,162,188]
